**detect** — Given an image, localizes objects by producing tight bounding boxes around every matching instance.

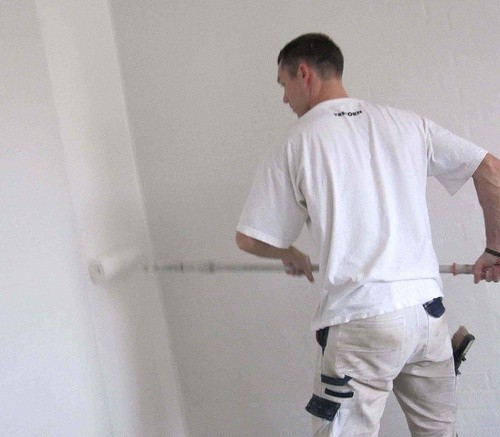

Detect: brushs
[88,240,500,284]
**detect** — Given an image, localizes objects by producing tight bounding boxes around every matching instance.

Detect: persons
[235,32,500,437]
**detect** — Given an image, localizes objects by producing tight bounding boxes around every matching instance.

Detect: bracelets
[485,248,500,257]
